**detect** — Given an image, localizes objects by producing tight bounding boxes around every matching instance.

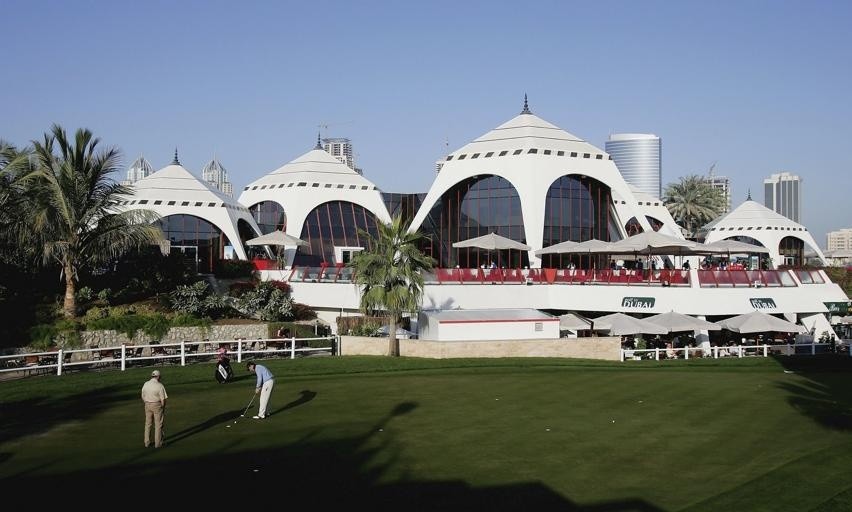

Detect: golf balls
[234,420,237,423]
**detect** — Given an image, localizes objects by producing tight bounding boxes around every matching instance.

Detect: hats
[151,370,160,377]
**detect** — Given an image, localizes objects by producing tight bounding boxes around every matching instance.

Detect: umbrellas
[641,308,722,348]
[594,312,669,337]
[245,229,310,270]
[715,309,801,345]
[535,229,770,269]
[452,231,532,269]
[558,313,611,330]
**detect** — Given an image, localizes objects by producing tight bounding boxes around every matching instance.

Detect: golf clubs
[241,387,261,417]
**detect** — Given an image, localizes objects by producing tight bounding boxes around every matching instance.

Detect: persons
[490,259,497,269]
[623,340,738,360]
[141,370,168,449]
[247,361,277,419]
[481,260,487,269]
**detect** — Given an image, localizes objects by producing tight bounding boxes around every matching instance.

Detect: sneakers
[252,413,270,419]
[144,442,168,449]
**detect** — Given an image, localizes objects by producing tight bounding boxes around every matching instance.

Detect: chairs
[0,337,289,377]
